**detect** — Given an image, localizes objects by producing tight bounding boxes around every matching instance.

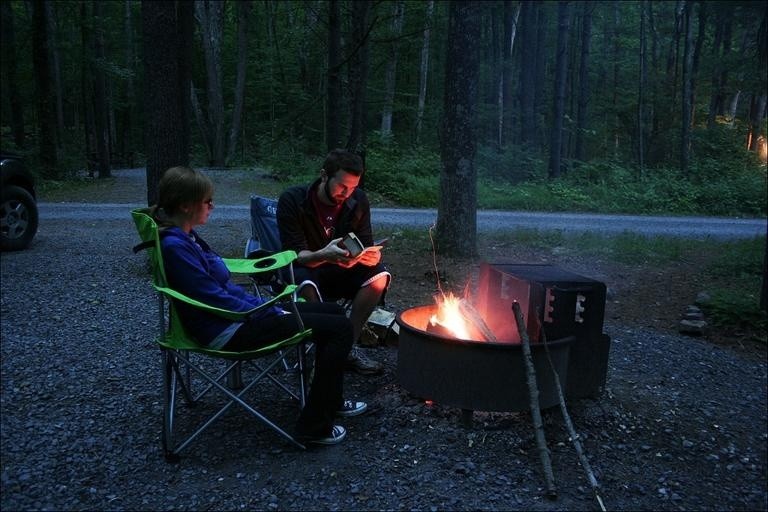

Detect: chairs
[129,204,316,463]
[243,196,358,370]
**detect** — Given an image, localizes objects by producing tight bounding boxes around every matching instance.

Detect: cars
[0,155,39,248]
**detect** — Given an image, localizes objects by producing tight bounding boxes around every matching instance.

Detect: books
[336,231,388,261]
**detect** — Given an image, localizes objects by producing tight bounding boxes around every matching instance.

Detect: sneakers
[344,347,384,376]
[335,400,368,417]
[298,425,346,445]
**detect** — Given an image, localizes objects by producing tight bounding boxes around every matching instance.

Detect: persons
[151,164,370,447]
[277,150,391,397]
